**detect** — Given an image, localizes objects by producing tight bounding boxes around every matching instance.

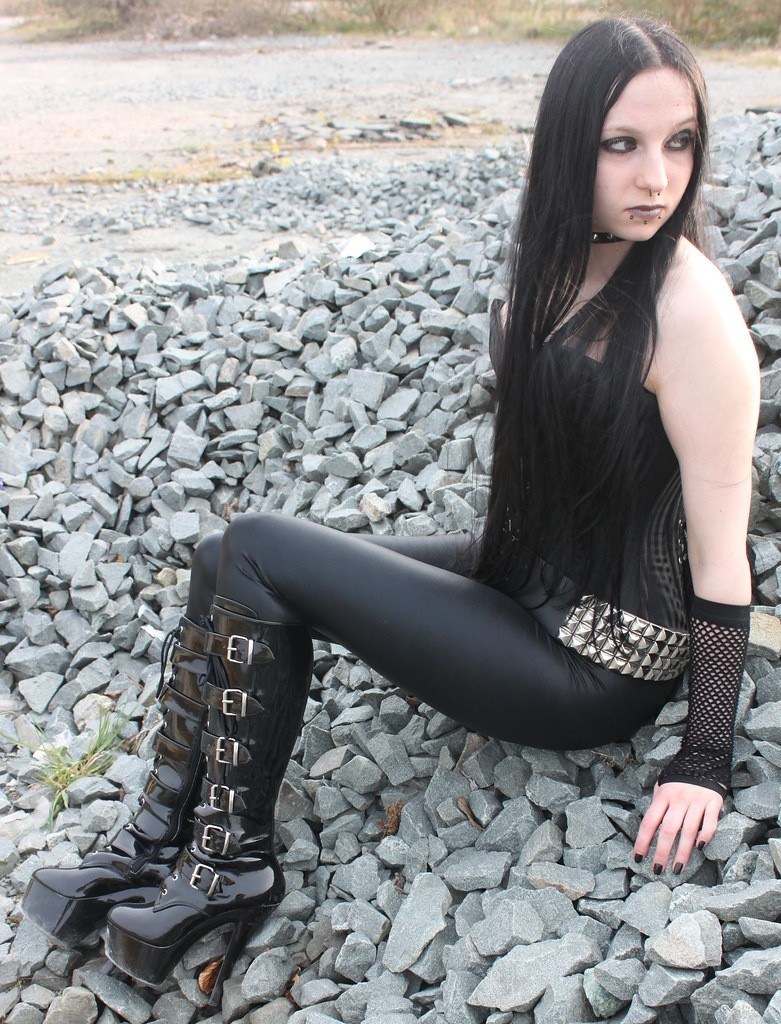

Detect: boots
[19,614,211,947]
[103,593,287,1008]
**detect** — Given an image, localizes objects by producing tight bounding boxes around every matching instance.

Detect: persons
[24,21,762,1009]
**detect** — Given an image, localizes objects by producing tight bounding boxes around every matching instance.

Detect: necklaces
[589,231,626,243]
